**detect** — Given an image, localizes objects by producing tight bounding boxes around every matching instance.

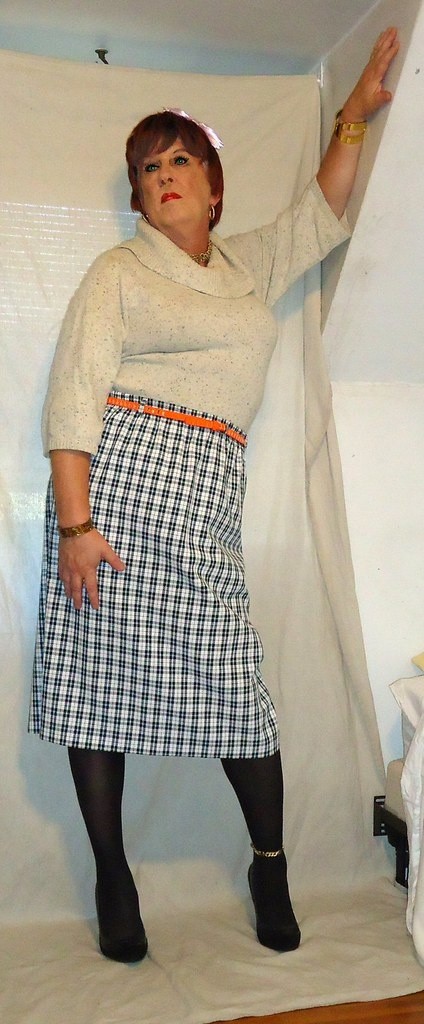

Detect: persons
[28,27,401,963]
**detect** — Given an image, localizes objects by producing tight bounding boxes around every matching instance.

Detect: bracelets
[333,129,365,144]
[335,110,367,131]
[58,521,95,540]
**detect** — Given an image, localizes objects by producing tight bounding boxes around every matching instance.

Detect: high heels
[246,847,300,953]
[93,862,148,962]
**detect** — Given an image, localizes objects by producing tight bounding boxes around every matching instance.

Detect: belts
[107,397,248,448]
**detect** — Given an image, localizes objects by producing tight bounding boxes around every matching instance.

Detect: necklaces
[188,241,211,263]
